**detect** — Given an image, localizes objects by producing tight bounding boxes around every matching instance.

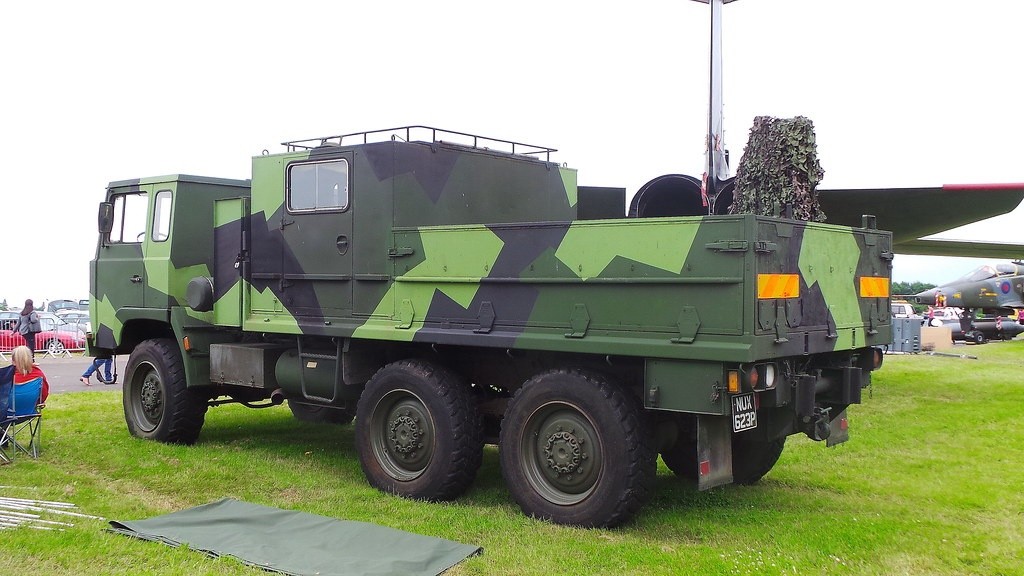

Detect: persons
[1018,309,1024,325]
[10,299,41,365]
[4,322,19,332]
[80,358,118,385]
[0,346,49,447]
[923,306,938,327]
[940,295,944,307]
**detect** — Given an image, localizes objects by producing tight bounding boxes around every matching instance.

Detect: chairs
[0,365,46,467]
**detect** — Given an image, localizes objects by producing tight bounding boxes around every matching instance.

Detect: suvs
[891,300,916,318]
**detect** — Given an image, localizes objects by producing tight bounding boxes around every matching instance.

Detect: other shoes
[80,377,93,385]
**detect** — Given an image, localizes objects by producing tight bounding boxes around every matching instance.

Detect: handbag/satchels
[28,311,42,333]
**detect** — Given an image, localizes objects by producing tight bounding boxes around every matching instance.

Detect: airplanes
[915,260,1024,310]
[578,0,1024,262]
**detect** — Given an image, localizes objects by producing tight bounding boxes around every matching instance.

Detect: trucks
[85,126,895,526]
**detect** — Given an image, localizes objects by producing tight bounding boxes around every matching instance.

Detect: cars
[0,298,93,354]
